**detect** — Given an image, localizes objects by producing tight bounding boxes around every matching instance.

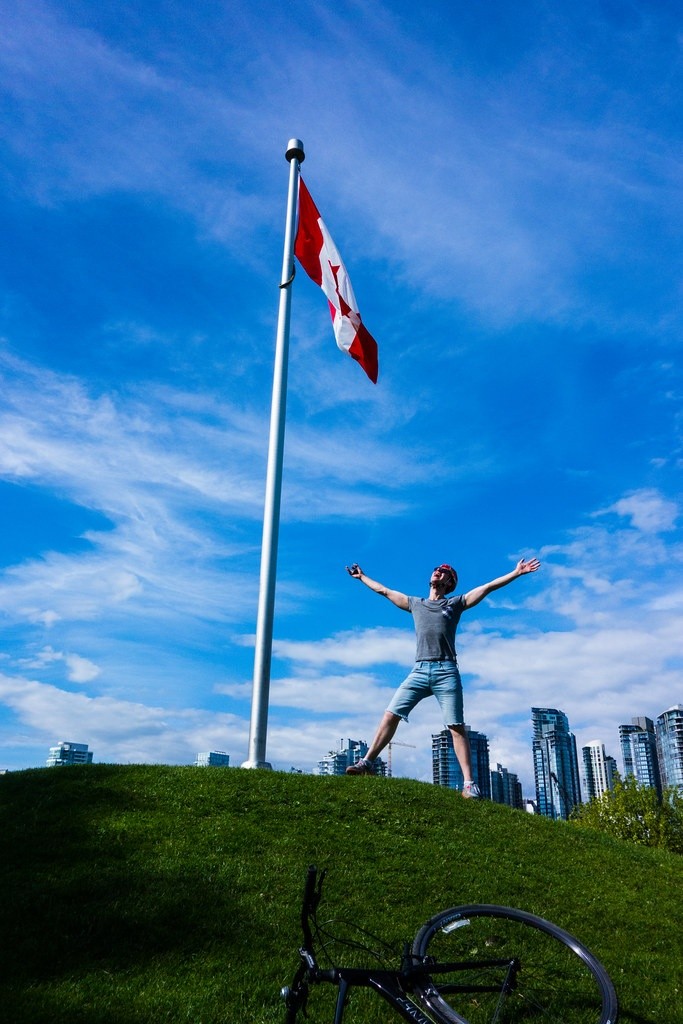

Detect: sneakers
[346,758,373,775]
[462,784,478,798]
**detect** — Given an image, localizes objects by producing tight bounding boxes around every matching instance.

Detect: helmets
[440,564,457,584]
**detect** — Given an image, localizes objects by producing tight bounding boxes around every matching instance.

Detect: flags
[293,175,379,386]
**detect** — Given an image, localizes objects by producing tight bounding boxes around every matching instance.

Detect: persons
[346,557,542,798]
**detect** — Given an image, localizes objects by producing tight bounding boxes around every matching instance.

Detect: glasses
[433,567,451,575]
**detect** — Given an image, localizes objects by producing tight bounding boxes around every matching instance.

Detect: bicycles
[277,862,622,1024]
